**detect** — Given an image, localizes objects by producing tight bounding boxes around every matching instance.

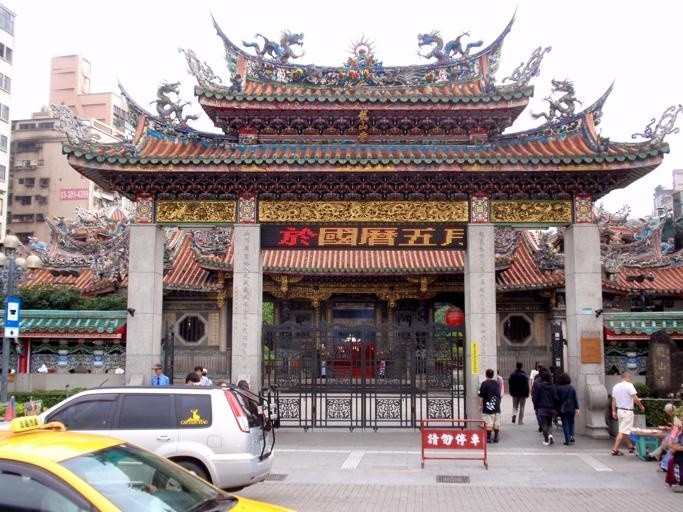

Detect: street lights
[0,235,42,404]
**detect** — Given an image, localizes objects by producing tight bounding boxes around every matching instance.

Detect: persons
[649,403,682,461]
[667,415,683,493]
[611,372,646,456]
[151,364,169,385]
[194,367,210,385]
[477,362,580,446]
[238,381,248,390]
[202,368,213,385]
[185,373,200,385]
[217,381,227,386]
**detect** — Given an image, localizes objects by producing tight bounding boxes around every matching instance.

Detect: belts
[617,407,634,412]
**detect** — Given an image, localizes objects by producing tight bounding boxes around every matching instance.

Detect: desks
[627,427,664,460]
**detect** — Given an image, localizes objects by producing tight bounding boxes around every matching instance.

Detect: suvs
[0,385,280,502]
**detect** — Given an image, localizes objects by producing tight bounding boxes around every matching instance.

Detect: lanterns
[445,305,465,326]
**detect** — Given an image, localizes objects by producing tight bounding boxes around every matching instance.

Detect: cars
[0,414,297,511]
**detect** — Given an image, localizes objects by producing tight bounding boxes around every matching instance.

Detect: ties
[157,377,160,385]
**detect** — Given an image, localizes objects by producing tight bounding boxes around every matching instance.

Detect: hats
[152,364,162,369]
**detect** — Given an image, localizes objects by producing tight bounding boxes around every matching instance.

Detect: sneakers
[542,434,555,446]
[488,437,498,444]
[564,438,576,445]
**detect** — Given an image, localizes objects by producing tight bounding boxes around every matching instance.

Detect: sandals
[628,445,638,454]
[611,449,624,456]
